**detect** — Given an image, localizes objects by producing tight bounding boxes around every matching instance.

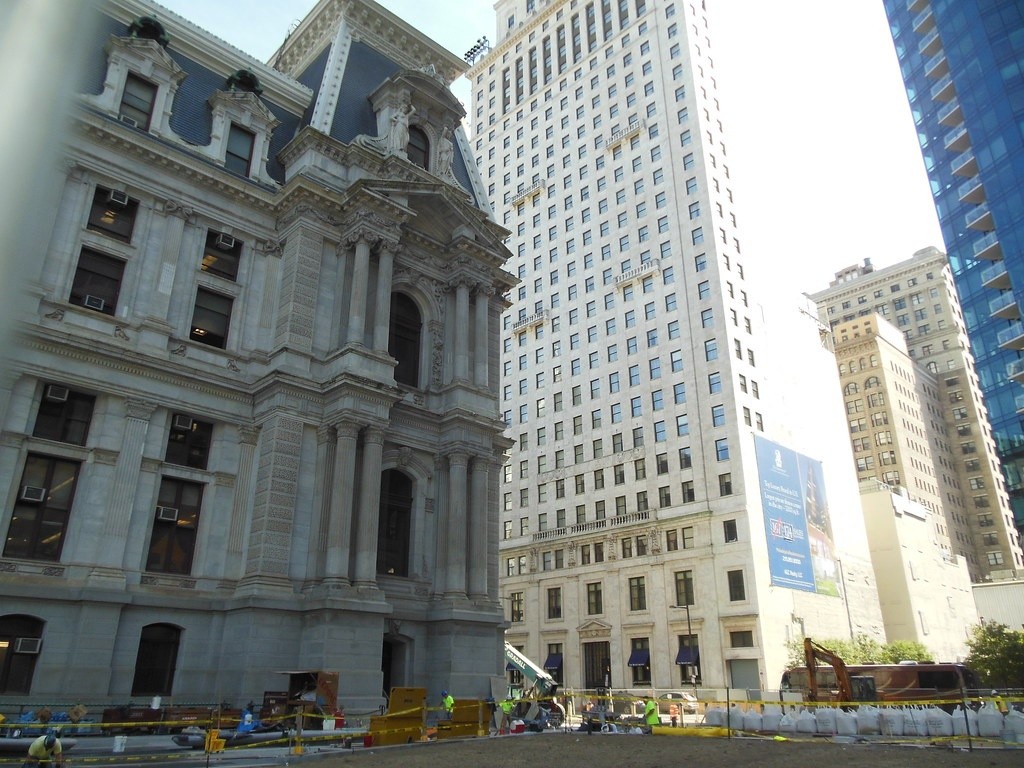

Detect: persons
[390,105,416,150]
[643,696,660,726]
[438,690,454,719]
[669,702,679,727]
[991,690,1009,716]
[438,127,455,175]
[24,734,62,768]
[496,695,514,728]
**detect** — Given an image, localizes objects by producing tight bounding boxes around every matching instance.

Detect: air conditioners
[20,485,46,503]
[106,190,128,206]
[216,234,234,250]
[157,506,178,522]
[173,415,192,429]
[45,385,68,401]
[120,115,137,129]
[13,637,42,653]
[83,295,105,311]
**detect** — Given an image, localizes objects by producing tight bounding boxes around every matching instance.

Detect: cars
[612,694,646,714]
[654,691,699,714]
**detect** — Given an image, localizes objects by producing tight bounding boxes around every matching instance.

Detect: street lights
[668,604,697,689]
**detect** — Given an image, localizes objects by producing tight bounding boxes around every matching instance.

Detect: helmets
[44,734,56,747]
[505,695,512,699]
[441,690,449,696]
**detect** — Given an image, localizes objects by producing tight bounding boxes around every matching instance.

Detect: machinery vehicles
[803,637,886,711]
[504,639,566,732]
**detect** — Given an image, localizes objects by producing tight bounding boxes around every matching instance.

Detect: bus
[780,661,982,715]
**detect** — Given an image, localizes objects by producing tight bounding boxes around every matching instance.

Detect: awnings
[544,653,562,671]
[675,646,699,666]
[506,663,518,671]
[628,649,649,667]
